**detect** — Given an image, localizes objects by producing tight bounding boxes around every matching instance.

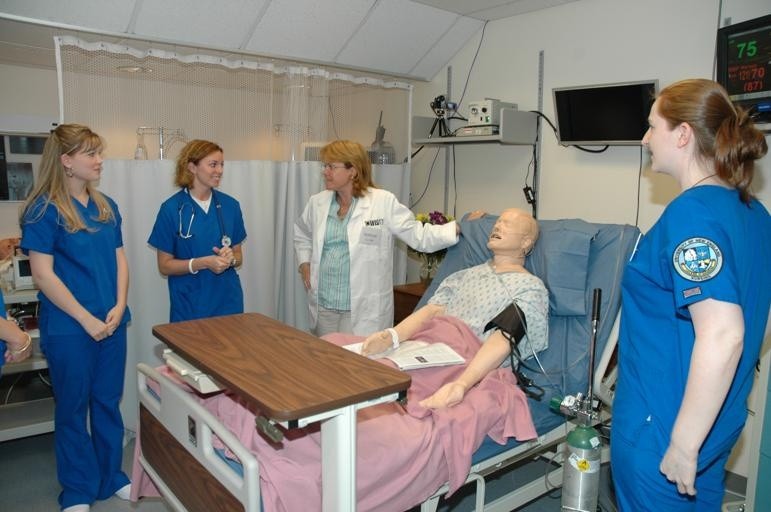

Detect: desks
[150,311,413,512]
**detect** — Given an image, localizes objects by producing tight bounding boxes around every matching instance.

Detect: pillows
[459,218,595,319]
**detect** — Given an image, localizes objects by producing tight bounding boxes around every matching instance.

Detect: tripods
[428,116,451,137]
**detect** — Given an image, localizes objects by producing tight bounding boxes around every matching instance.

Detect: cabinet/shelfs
[0,289,56,442]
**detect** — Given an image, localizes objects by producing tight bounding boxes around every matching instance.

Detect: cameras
[429,94,456,116]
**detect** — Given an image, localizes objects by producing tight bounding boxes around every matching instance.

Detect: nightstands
[393,282,428,328]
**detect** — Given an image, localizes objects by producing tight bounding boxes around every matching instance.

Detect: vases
[417,260,439,287]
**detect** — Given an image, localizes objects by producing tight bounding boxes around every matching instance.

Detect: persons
[610,78,771,512]
[292,140,462,335]
[146,138,247,322]
[16,122,133,512]
[0,289,33,376]
[360,208,550,409]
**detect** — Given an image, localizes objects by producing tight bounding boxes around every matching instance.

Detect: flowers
[404,212,452,265]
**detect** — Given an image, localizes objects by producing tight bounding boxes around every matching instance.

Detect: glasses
[320,163,346,171]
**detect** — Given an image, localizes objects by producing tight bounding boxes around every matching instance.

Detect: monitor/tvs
[551,78,659,145]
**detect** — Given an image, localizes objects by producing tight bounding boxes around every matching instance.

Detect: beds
[133,213,644,511]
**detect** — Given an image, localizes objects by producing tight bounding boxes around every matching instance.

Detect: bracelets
[11,331,32,353]
[189,258,200,275]
